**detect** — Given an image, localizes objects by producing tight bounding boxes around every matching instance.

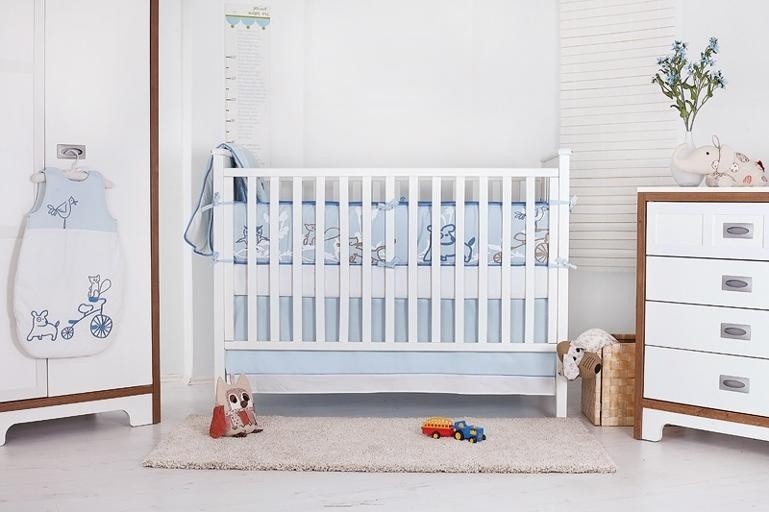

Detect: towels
[185,143,270,256]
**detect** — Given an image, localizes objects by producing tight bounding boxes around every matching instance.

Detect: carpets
[143,413,619,474]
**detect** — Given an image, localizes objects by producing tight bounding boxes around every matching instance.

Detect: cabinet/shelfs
[633,186,769,444]
[0,0,160,448]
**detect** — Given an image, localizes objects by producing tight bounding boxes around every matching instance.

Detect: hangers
[30,148,116,189]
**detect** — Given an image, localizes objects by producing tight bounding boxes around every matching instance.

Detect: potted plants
[650,33,729,187]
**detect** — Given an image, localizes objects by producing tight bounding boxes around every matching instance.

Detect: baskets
[579,331,682,428]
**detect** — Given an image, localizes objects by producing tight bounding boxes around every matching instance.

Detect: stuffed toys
[208,373,264,438]
[686,141,768,188]
[554,327,618,381]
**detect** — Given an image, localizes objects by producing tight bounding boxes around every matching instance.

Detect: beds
[209,147,574,417]
